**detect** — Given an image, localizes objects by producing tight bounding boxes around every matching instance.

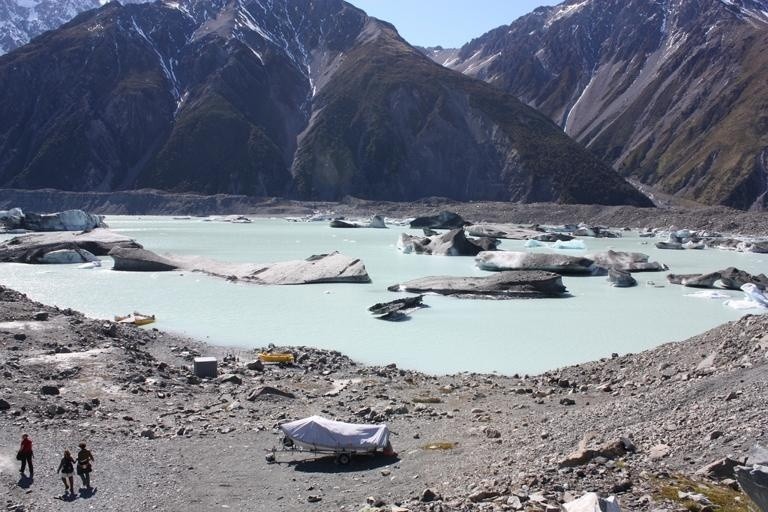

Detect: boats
[258,350,294,363]
[114,312,155,325]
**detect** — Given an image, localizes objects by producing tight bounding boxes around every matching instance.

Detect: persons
[76,442,95,490]
[18,433,34,478]
[56,450,78,493]
